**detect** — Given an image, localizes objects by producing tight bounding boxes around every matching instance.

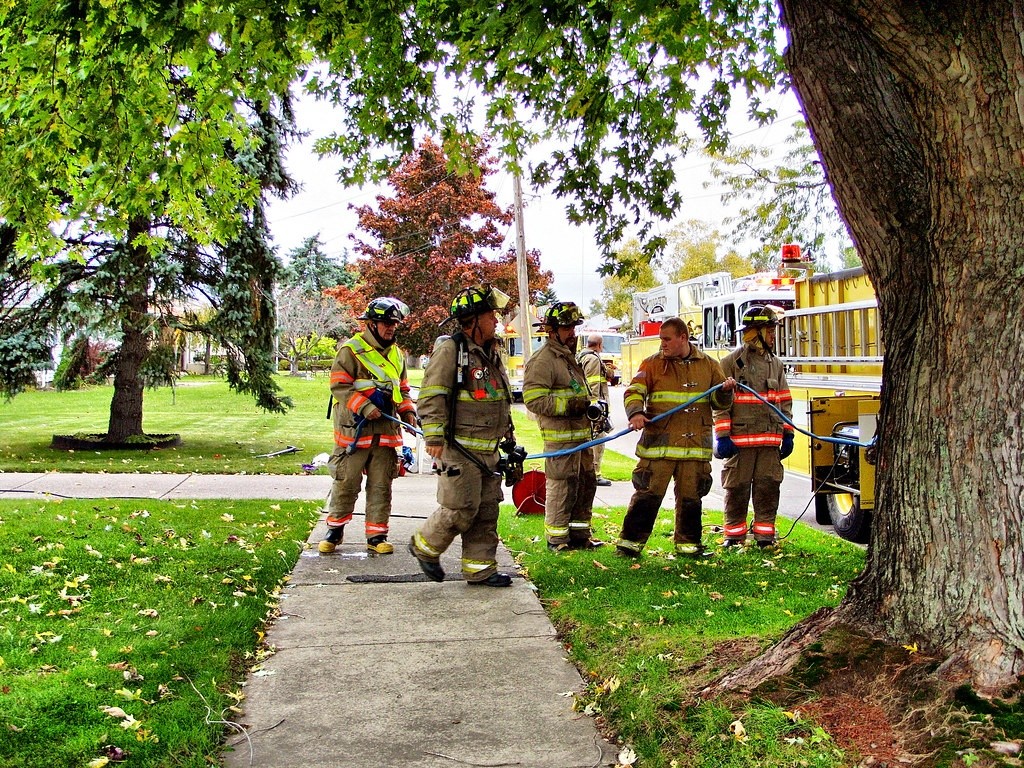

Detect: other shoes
[616,546,641,559]
[678,548,714,559]
[318,524,345,553]
[757,541,774,549]
[366,534,394,553]
[597,479,612,486]
[721,540,747,547]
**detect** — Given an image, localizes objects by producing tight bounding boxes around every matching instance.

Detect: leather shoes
[409,535,446,583]
[467,571,514,587]
[568,538,606,550]
[546,541,570,553]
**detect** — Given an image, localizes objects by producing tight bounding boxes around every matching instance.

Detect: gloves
[779,433,795,460]
[716,436,739,458]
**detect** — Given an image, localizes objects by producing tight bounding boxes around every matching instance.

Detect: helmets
[734,307,778,332]
[437,281,510,328]
[532,301,585,328]
[354,296,411,324]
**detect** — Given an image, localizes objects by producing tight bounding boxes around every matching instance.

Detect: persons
[522,302,604,551]
[616,317,736,559]
[408,287,516,588]
[712,307,795,555]
[576,333,612,487]
[318,297,418,553]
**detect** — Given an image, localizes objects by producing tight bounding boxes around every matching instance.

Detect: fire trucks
[621,244,884,545]
[498,325,624,403]
